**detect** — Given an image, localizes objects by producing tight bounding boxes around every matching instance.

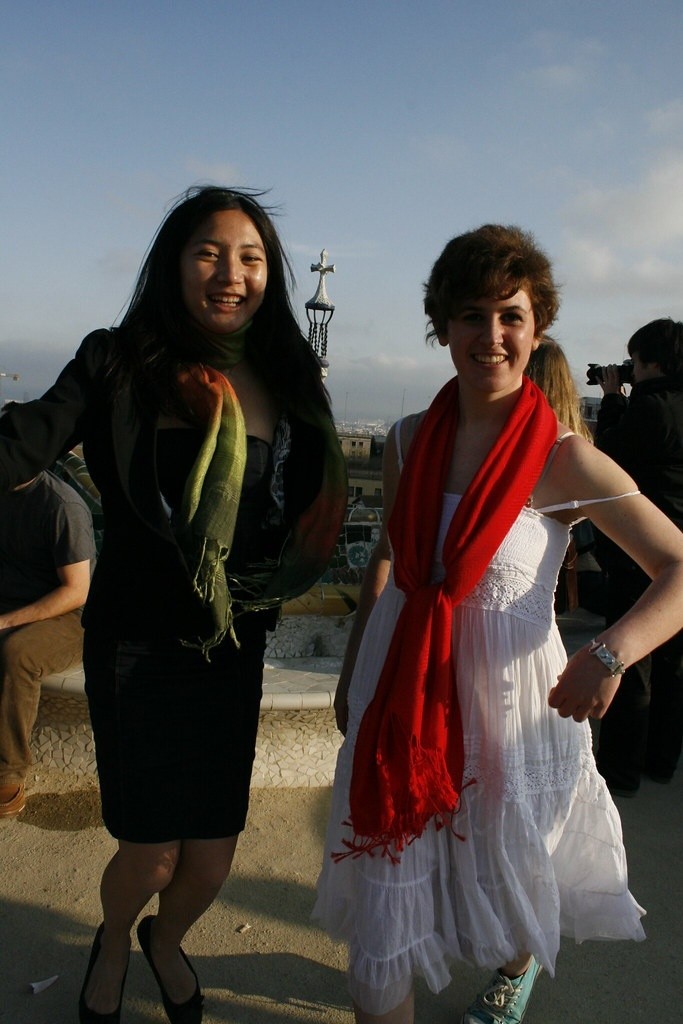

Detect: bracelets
[589,637,626,678]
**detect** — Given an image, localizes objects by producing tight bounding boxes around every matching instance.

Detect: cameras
[587,359,634,385]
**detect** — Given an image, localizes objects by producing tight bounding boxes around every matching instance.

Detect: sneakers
[460,953,542,1024]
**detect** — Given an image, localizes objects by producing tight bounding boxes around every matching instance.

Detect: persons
[524,319,683,795]
[0,471,98,817]
[309,225,682,1024]
[0,189,344,1024]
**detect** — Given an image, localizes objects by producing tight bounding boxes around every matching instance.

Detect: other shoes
[0,782,26,815]
[597,767,640,797]
[78,921,132,1024]
[639,765,672,784]
[137,914,204,1024]
